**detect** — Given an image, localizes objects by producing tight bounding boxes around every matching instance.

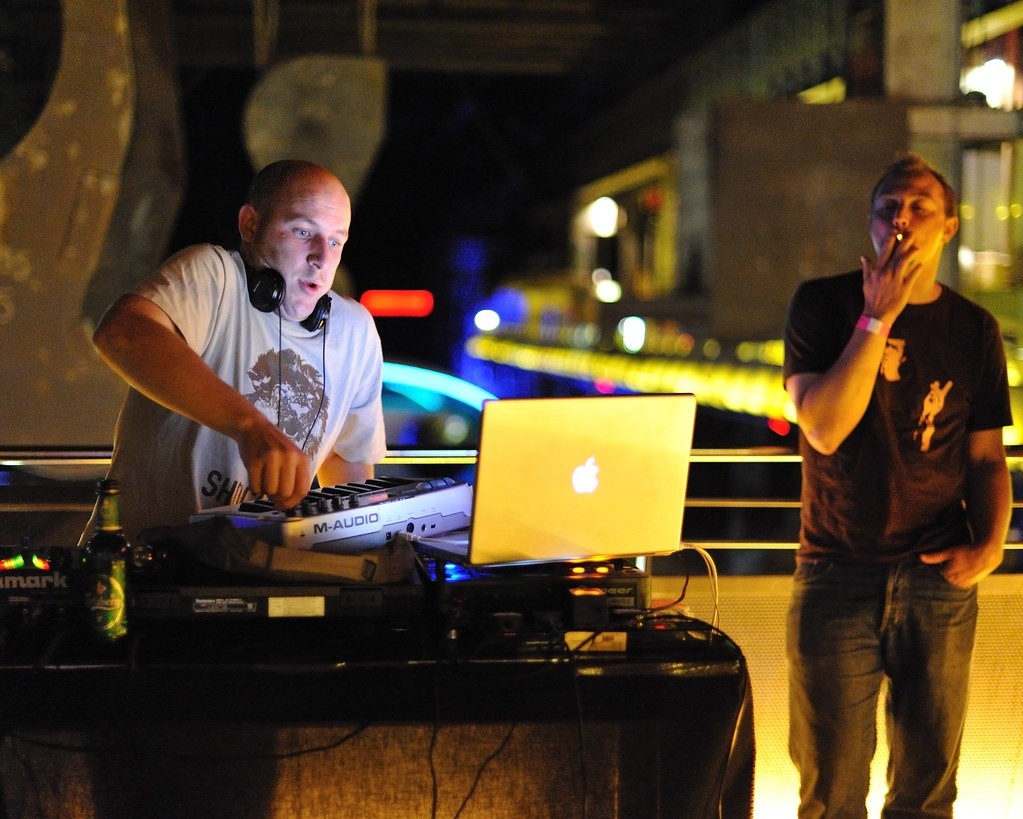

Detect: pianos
[180,471,475,556]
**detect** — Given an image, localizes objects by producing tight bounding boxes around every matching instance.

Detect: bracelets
[855,314,890,339]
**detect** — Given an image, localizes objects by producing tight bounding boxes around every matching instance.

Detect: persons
[782,157,1013,819]
[77,161,384,819]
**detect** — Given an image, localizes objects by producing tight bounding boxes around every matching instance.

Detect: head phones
[244,266,333,332]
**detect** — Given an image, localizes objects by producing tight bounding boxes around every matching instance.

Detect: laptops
[413,391,698,568]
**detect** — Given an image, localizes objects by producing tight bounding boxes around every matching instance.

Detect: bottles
[82,480,131,660]
